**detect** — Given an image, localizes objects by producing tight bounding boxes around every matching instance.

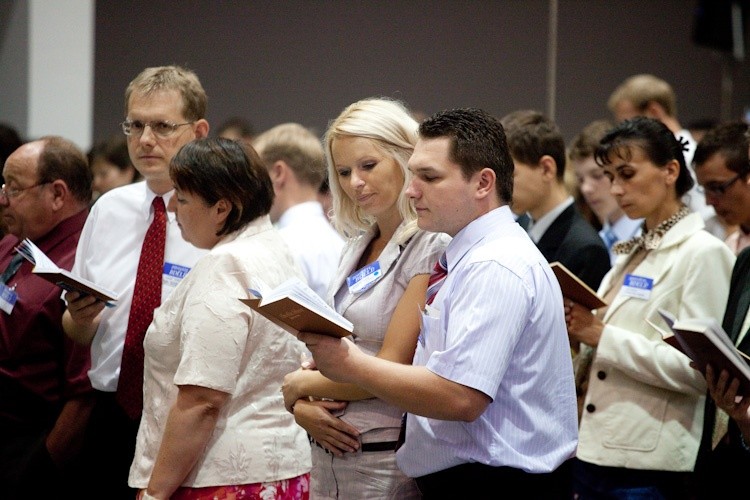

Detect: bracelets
[141,490,157,500]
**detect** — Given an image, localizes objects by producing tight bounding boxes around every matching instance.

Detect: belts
[307,434,397,453]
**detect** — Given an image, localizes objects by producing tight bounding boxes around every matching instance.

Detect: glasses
[2,180,52,196]
[697,174,741,196]
[119,119,194,137]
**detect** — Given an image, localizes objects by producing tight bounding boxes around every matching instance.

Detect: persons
[0,68,750,500]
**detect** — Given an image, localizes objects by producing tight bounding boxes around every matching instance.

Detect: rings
[569,302,574,309]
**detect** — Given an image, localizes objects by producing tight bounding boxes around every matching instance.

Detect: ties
[602,230,618,249]
[0,248,30,285]
[422,252,447,312]
[116,197,168,422]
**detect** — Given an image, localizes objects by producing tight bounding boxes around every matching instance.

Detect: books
[237,275,354,339]
[645,308,750,396]
[549,262,608,312]
[14,238,119,303]
